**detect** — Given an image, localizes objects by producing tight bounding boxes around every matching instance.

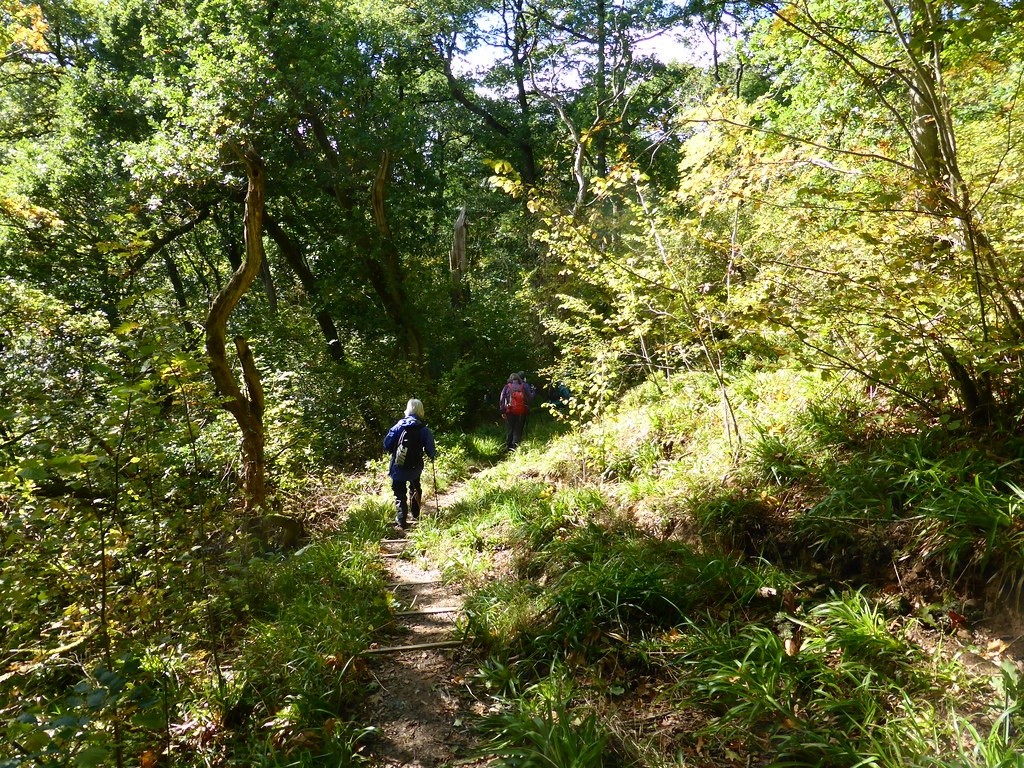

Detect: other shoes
[395,516,407,528]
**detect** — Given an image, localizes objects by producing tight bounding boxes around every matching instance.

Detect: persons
[500,373,534,453]
[548,382,570,411]
[517,371,535,438]
[383,399,436,527]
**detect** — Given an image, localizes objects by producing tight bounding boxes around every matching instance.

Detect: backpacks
[507,384,528,415]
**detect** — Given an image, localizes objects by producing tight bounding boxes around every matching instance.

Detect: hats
[518,371,525,378]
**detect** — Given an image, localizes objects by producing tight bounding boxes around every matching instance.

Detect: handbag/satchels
[395,430,409,466]
